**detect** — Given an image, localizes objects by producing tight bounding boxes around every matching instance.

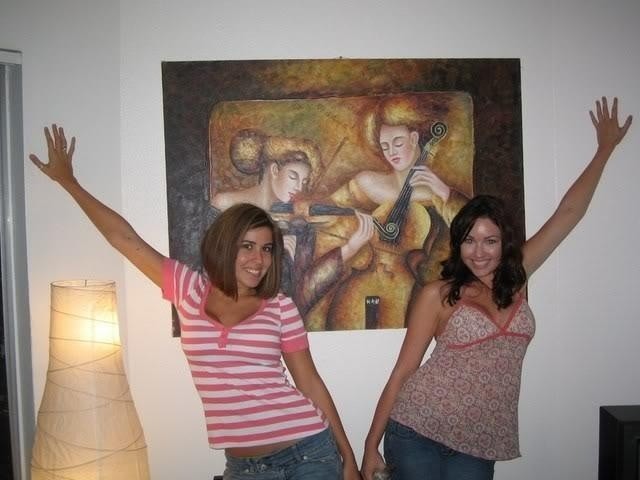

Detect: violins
[269,194,399,240]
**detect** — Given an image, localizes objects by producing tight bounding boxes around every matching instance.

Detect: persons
[29,121,359,480]
[322,88,474,332]
[207,128,376,315]
[359,94,634,480]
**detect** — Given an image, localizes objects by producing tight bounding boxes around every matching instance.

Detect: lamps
[29,276,149,478]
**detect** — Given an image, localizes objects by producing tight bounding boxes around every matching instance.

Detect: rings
[63,146,68,152]
[40,164,43,170]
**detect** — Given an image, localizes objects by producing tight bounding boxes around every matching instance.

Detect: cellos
[325,121,448,331]
